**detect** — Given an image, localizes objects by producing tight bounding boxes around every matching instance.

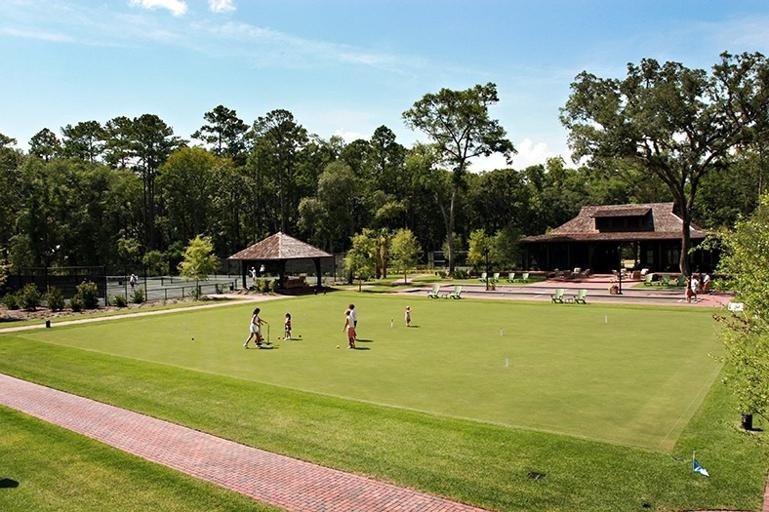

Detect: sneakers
[283,336,291,340]
[346,337,356,349]
[243,343,261,349]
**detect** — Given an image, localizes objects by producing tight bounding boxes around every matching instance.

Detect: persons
[348,304,358,340]
[251,312,265,342]
[282,312,292,339]
[686,276,701,303]
[128,273,137,291]
[244,307,268,349]
[249,265,257,281]
[405,305,417,327]
[341,310,355,349]
[259,264,266,277]
[701,272,713,295]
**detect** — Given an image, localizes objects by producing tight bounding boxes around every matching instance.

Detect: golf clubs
[267,323,272,345]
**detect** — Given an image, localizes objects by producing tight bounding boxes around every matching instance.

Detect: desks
[442,294,448,299]
[565,298,573,303]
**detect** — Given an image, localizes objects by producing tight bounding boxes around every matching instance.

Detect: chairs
[554,268,591,280]
[550,288,564,303]
[427,285,440,298]
[574,288,588,304]
[478,273,530,283]
[612,268,649,279]
[450,286,463,299]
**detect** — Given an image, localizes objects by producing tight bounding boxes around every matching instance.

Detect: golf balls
[278,337,280,339]
[299,335,301,337]
[192,338,194,340]
[336,345,339,349]
